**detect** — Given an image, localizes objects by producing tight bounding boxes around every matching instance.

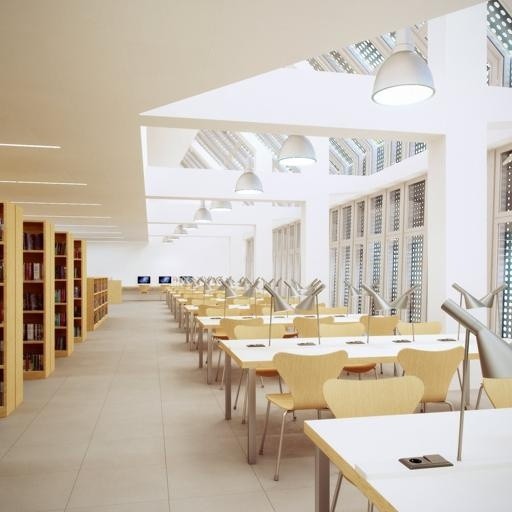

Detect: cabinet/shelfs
[0,201,87,415]
[87,275,110,332]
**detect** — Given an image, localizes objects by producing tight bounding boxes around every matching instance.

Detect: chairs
[321,375,428,512]
[161,278,512,482]
[138,285,151,296]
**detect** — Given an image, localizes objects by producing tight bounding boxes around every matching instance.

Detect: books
[22,232,44,371]
[55,241,67,351]
[74,246,82,338]
[0,221,5,408]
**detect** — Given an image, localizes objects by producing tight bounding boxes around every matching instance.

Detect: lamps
[371,28,436,105]
[182,224,198,231]
[193,200,212,224]
[174,224,188,235]
[277,134,317,169]
[208,200,233,213]
[234,155,264,196]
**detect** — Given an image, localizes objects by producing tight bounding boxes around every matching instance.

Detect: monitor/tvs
[138,276,150,284]
[159,276,171,283]
[180,276,193,283]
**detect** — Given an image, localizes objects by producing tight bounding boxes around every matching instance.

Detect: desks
[304,407,511,512]
